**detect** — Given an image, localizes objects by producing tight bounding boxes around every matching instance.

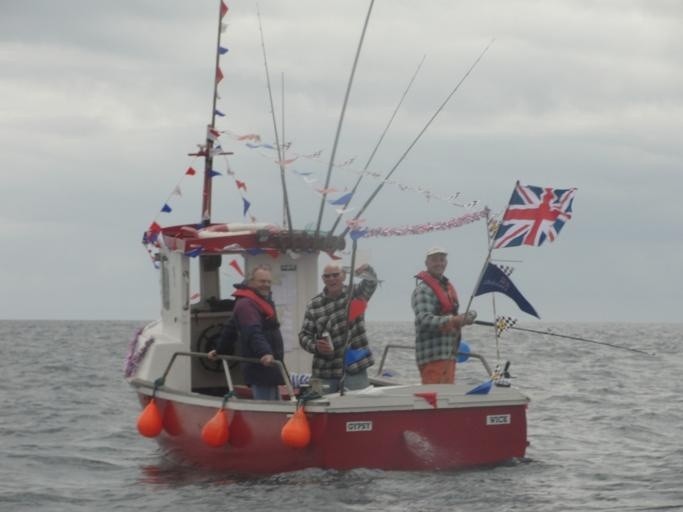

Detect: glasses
[324,272,339,277]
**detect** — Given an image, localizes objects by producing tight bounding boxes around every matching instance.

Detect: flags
[491,183,576,247]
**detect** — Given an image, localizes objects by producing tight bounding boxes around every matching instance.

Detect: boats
[122,216,534,475]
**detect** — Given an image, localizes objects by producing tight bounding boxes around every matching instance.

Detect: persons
[297,259,376,394]
[410,248,477,385]
[223,264,290,400]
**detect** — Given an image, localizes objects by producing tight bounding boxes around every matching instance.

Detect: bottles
[322,328,334,352]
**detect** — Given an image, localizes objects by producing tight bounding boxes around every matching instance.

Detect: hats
[427,247,448,256]
